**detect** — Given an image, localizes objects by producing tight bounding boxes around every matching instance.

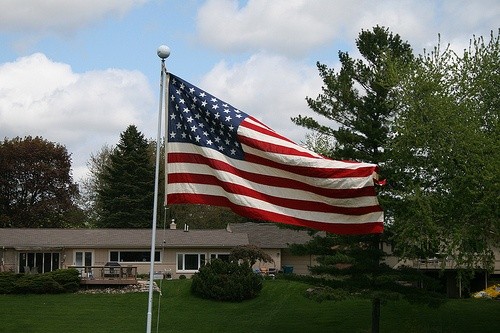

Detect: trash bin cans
[284,264,293,273]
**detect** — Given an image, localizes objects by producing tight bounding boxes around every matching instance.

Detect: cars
[101,262,123,278]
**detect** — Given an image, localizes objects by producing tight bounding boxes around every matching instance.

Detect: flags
[165,71,387,234]
